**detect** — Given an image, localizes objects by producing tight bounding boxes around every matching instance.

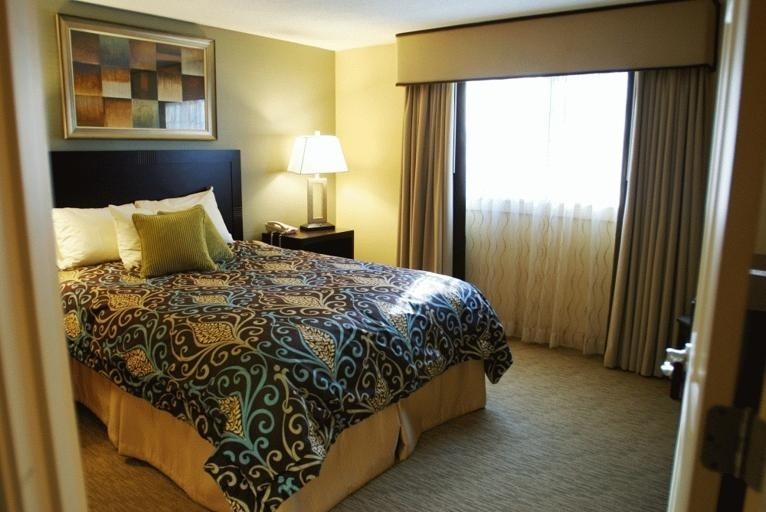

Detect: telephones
[265,221,299,235]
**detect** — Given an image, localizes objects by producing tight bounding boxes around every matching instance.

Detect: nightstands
[261,229,354,260]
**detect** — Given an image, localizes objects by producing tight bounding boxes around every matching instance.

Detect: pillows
[51,186,237,280]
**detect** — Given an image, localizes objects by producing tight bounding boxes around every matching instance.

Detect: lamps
[287,130,350,232]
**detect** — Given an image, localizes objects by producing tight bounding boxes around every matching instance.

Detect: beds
[49,149,513,512]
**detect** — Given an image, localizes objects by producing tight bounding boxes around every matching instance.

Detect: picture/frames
[56,12,217,140]
